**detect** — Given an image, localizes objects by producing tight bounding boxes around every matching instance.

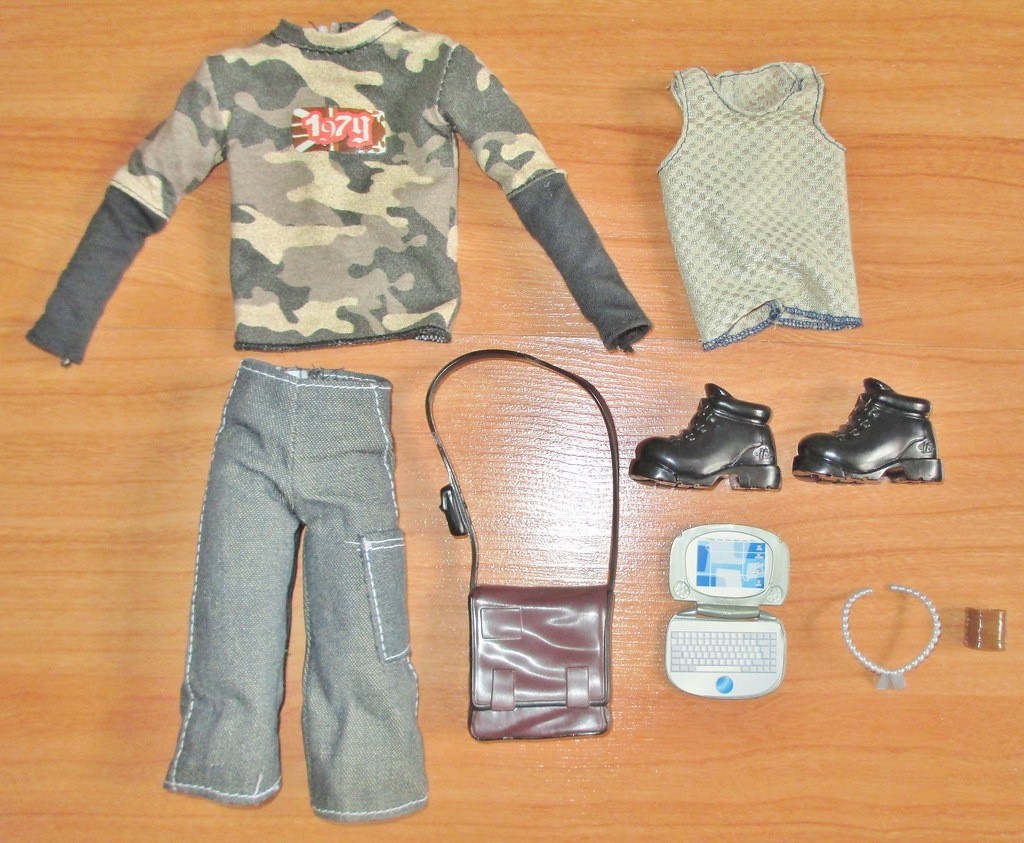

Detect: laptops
[663,522,791,701]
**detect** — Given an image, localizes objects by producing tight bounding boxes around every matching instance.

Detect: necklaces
[842,586,941,693]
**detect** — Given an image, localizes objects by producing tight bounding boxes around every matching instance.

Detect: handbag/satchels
[467,586,613,741]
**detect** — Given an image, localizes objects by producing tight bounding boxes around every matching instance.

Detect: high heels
[628,383,781,490]
[792,377,942,484]
